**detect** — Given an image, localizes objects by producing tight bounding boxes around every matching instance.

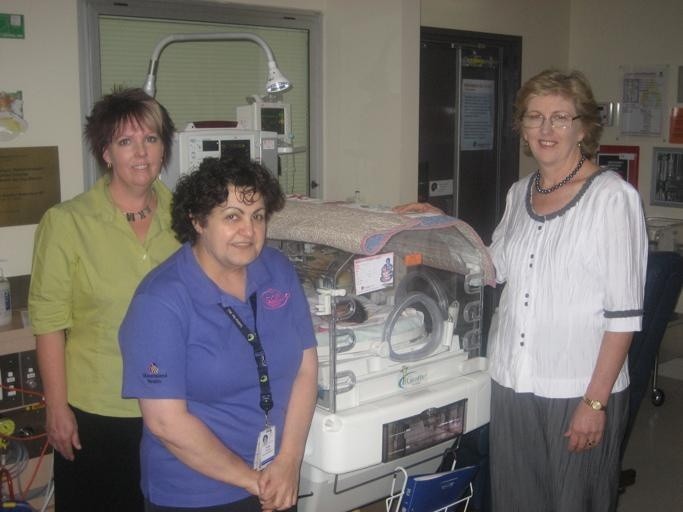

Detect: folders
[398,463,480,511]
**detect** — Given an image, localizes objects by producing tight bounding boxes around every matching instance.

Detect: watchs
[582,395,607,412]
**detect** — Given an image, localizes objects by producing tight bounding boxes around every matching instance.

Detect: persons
[262,435,269,446]
[116,149,319,512]
[25,83,183,510]
[393,67,651,512]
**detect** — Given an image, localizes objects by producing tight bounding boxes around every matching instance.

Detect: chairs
[612,248,681,494]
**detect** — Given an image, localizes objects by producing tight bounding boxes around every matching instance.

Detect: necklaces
[122,186,155,222]
[534,154,587,194]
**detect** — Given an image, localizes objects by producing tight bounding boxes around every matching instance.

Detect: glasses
[520,109,583,131]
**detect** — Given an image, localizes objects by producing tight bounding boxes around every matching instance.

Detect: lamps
[142,31,291,100]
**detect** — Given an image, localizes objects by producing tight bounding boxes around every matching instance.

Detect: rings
[587,439,596,447]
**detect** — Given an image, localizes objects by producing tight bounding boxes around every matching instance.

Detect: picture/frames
[648,147,682,209]
